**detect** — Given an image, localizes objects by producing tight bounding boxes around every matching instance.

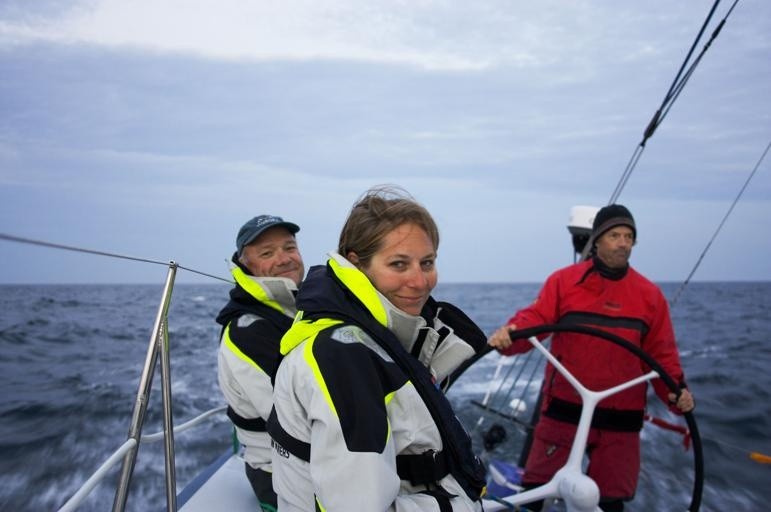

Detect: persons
[216,214,307,511]
[488,205,696,512]
[263,184,488,511]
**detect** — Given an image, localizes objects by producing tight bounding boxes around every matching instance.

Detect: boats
[0,1,770,512]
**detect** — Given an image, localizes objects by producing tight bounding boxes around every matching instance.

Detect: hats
[591,204,636,250]
[236,215,299,257]
